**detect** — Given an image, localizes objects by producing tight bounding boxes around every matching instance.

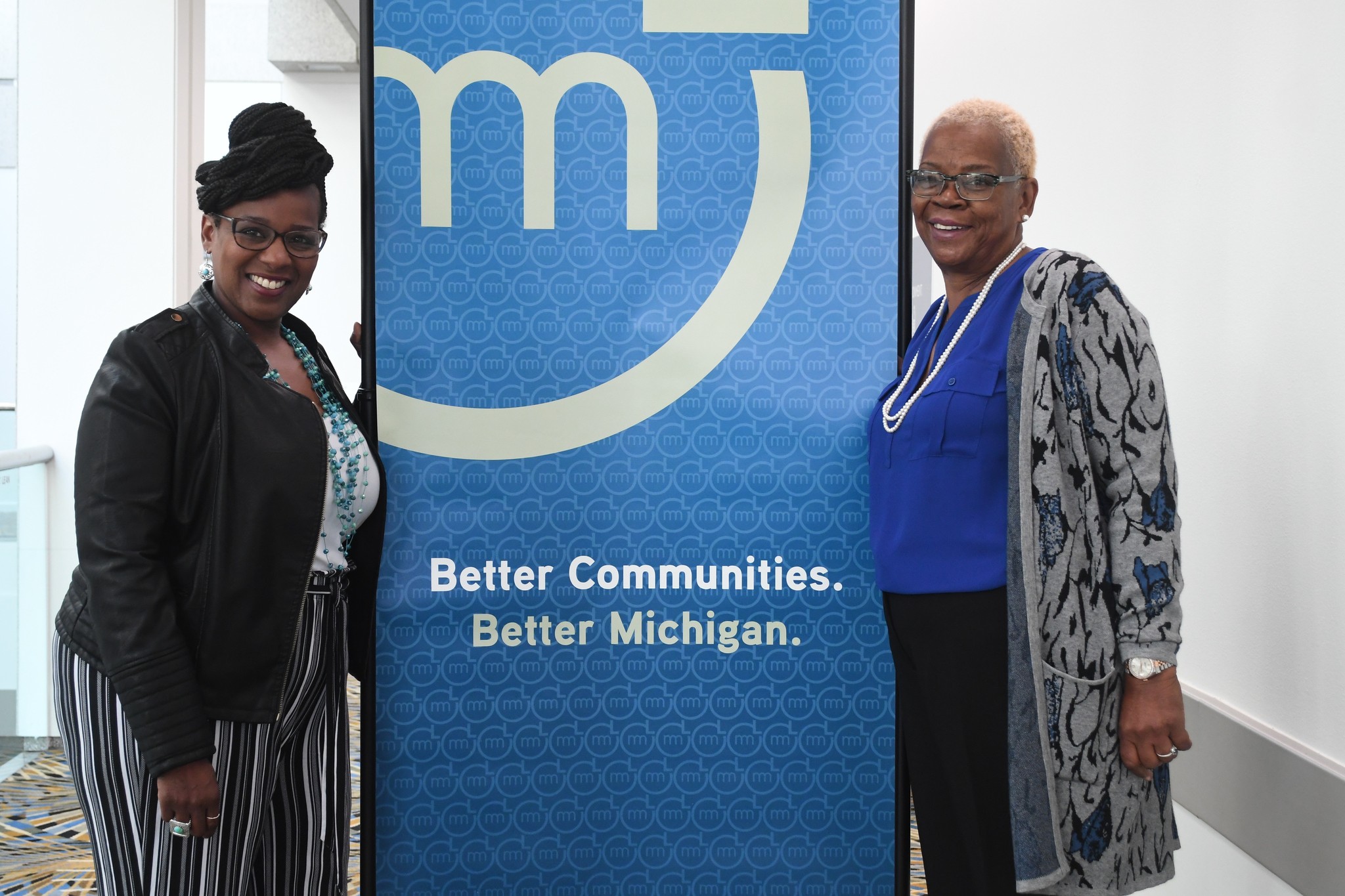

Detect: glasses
[209,211,328,259]
[906,170,1028,200]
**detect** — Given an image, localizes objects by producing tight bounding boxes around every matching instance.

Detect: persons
[866,98,1195,896]
[48,99,384,896]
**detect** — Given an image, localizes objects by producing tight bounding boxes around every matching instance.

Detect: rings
[1155,744,1178,758]
[168,815,192,837]
[205,814,220,819]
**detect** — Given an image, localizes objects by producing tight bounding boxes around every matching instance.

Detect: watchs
[1126,657,1175,682]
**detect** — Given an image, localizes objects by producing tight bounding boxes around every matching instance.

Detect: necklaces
[881,240,1026,434]
[234,323,369,570]
